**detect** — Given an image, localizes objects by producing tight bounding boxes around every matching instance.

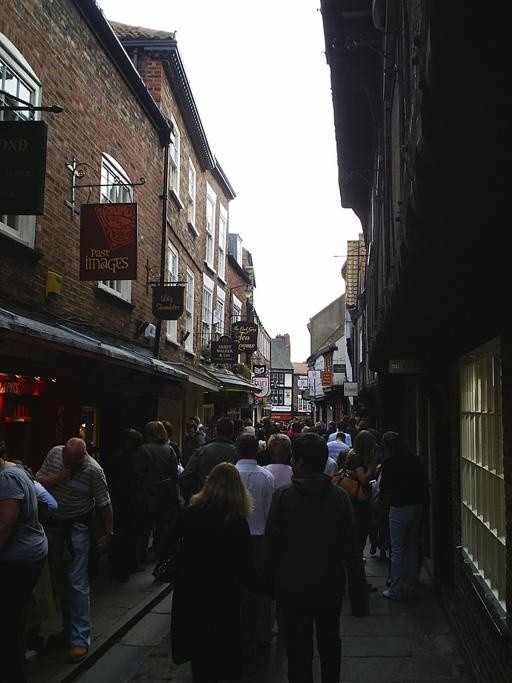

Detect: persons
[167,462,250,683]
[261,434,358,683]
[0,439,115,666]
[108,414,421,658]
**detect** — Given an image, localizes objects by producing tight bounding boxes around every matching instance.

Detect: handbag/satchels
[142,472,177,507]
[331,468,371,504]
[153,536,184,584]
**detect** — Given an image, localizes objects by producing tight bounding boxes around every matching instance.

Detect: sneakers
[66,645,88,662]
